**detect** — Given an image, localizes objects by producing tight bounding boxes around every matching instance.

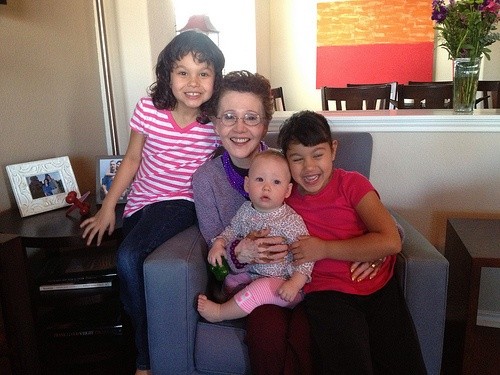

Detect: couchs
[143,131,450,375]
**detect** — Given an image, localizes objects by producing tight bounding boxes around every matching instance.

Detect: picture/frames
[5,155,81,217]
[96,155,132,204]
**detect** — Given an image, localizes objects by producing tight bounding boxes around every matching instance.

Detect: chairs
[322,81,500,110]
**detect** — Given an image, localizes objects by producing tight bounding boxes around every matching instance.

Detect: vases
[452,58,481,114]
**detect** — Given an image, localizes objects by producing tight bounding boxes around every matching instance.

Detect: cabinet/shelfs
[443,219,500,375]
[0,195,137,375]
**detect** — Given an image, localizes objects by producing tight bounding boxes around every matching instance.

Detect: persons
[196,148,315,323]
[276,110,428,375]
[79,30,225,375]
[190,70,405,375]
[29,160,132,200]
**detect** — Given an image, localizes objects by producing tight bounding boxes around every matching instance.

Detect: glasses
[214,112,268,126]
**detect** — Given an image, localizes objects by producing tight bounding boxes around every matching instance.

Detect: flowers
[431,0,500,61]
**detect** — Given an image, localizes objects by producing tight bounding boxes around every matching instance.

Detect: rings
[371,264,376,270]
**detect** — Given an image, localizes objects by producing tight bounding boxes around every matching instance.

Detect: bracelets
[230,239,245,270]
